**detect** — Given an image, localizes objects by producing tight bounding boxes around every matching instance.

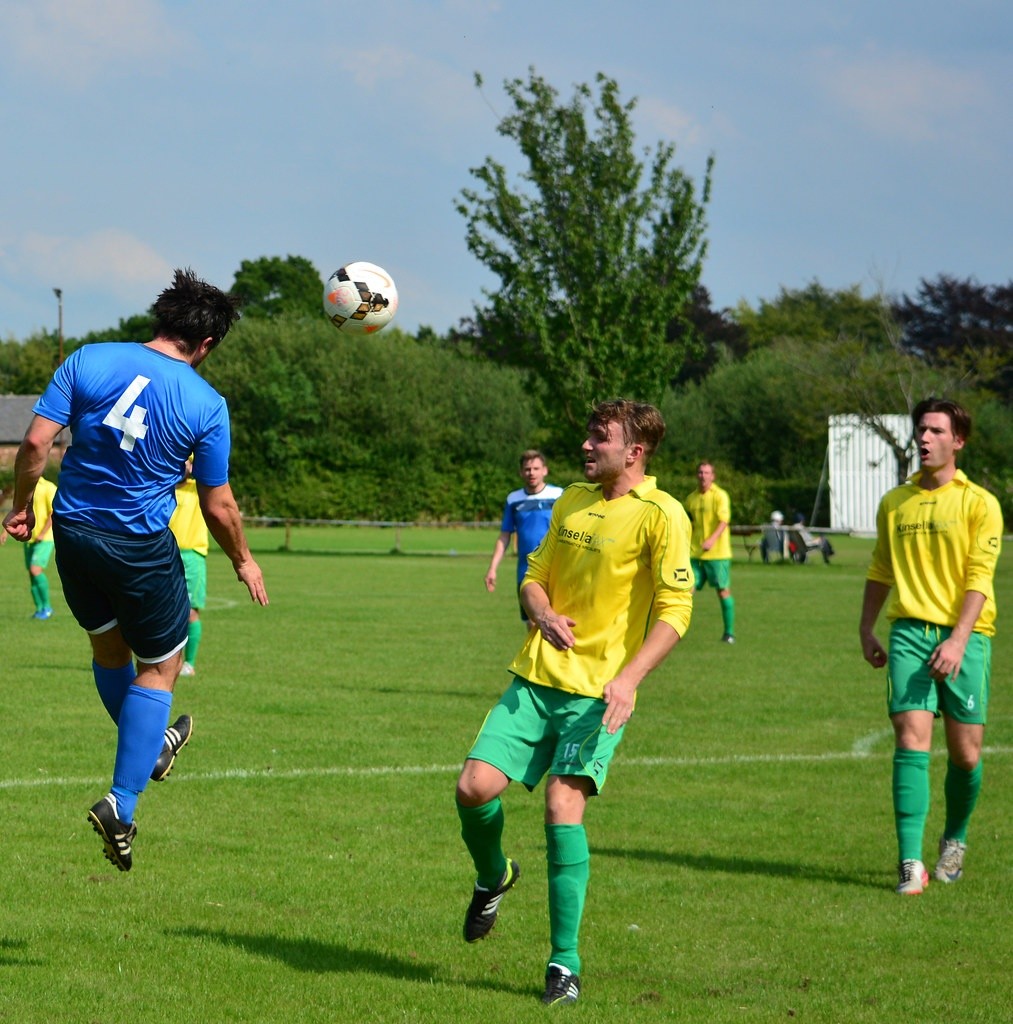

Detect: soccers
[321,260,399,338]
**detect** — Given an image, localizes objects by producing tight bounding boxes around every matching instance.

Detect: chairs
[761,527,819,562]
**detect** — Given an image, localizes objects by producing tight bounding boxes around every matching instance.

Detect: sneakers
[895,858,930,895]
[722,634,734,644]
[934,837,965,885]
[150,715,194,783]
[86,792,137,873]
[463,857,520,942]
[538,961,582,1009]
[32,608,53,620]
[180,662,195,677]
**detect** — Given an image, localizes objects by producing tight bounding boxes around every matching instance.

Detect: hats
[770,510,784,522]
[793,513,806,522]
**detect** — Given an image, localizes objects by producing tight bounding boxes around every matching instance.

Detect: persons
[683,461,735,643]
[483,451,563,630]
[859,401,1004,894]
[168,455,208,676]
[760,511,784,562]
[2,269,268,871]
[455,400,696,1003]
[793,513,834,564]
[1,474,57,619]
